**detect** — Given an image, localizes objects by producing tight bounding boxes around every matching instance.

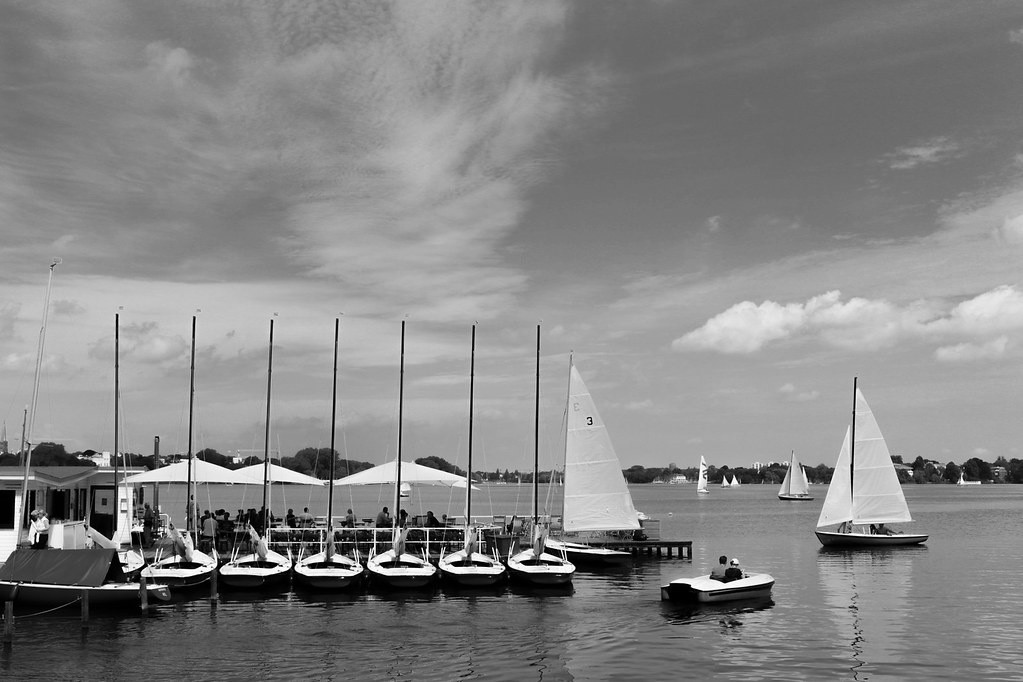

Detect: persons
[376,506,392,528]
[142,503,155,548]
[30,509,49,550]
[298,507,314,528]
[236,506,276,532]
[400,509,408,527]
[286,509,298,528]
[870,523,895,534]
[423,511,440,528]
[346,509,357,527]
[186,495,200,528]
[711,555,728,578]
[200,509,235,549]
[725,558,742,579]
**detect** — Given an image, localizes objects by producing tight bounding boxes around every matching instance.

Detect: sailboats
[506,324,579,585]
[138,315,220,593]
[720,475,741,488]
[216,320,295,590]
[293,317,367,587]
[439,324,506,584]
[539,348,648,568]
[809,376,931,549]
[776,449,816,502]
[364,321,434,587]
[696,455,718,495]
[0,260,170,607]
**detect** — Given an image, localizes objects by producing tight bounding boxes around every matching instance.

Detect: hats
[730,558,740,565]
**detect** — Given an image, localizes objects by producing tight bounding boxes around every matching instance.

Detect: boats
[658,569,776,605]
